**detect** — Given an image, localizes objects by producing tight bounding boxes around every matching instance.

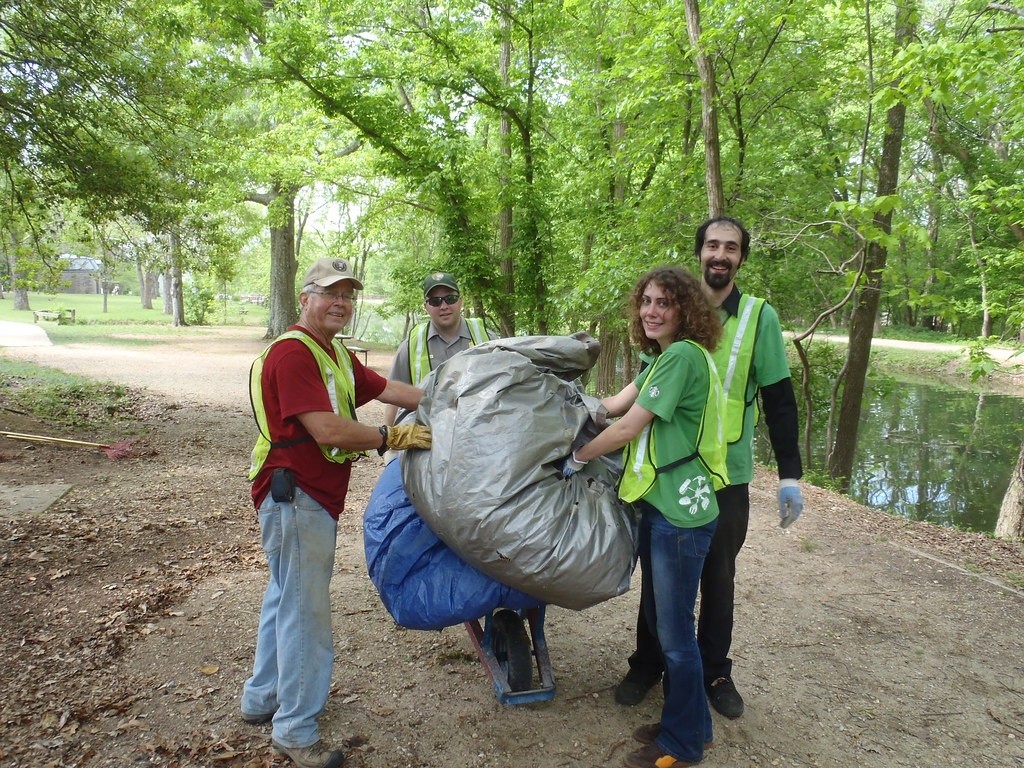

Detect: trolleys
[466,606,555,706]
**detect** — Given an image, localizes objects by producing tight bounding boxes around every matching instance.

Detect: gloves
[378,425,432,451]
[562,445,588,478]
[777,477,804,528]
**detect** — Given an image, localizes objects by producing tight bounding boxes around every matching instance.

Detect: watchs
[380,425,387,447]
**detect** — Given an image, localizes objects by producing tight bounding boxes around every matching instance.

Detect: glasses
[424,294,459,307]
[310,290,357,304]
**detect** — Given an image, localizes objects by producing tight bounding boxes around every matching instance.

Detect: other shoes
[633,722,712,750]
[614,665,664,705]
[705,671,744,719]
[623,745,700,768]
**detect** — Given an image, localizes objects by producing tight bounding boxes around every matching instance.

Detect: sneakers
[243,712,274,724]
[272,737,344,768]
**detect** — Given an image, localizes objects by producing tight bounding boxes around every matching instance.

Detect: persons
[562,268,730,768]
[613,217,804,718]
[381,272,501,465]
[240,258,431,768]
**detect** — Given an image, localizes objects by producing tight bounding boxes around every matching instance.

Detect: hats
[304,258,364,292]
[424,273,459,297]
